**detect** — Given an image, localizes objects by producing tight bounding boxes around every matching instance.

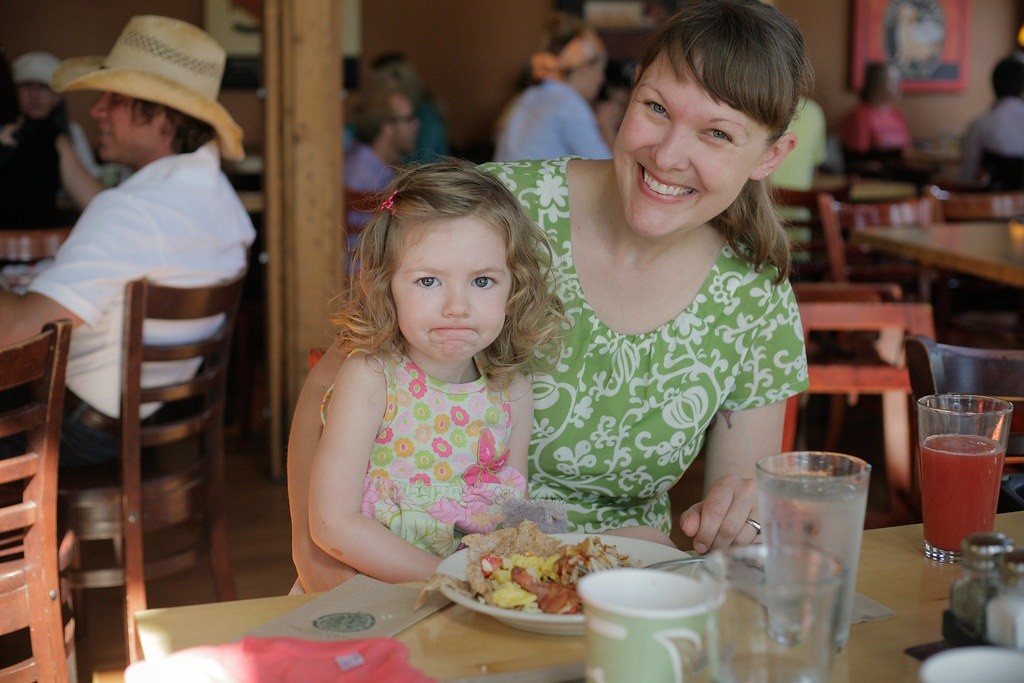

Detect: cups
[755,452,870,650]
[916,645,1023,682]
[698,542,840,682]
[917,394,1014,563]
[576,566,725,683]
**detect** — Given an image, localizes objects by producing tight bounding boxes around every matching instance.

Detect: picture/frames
[852,0,970,94]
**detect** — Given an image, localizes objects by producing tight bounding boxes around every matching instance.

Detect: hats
[13,51,58,84]
[53,14,244,161]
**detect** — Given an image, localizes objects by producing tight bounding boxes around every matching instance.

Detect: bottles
[983,549,1024,649]
[949,534,1014,636]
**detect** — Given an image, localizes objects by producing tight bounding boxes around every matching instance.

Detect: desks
[136,504,1024,683]
[857,214,1024,288]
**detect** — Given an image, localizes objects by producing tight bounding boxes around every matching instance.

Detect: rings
[746,517,762,534]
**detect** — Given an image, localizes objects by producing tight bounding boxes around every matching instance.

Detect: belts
[68,389,123,433]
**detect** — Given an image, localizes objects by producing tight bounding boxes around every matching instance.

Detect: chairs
[72,273,256,683]
[747,146,1024,510]
[0,318,80,683]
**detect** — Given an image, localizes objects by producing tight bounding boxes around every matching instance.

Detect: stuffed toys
[502,498,570,534]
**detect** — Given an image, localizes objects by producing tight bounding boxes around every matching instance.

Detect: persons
[959,59,1024,188]
[343,52,446,282]
[0,16,257,473]
[287,0,815,596]
[308,156,572,583]
[0,51,107,266]
[496,26,826,279]
[841,62,935,203]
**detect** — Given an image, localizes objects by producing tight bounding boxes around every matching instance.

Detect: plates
[434,532,726,636]
